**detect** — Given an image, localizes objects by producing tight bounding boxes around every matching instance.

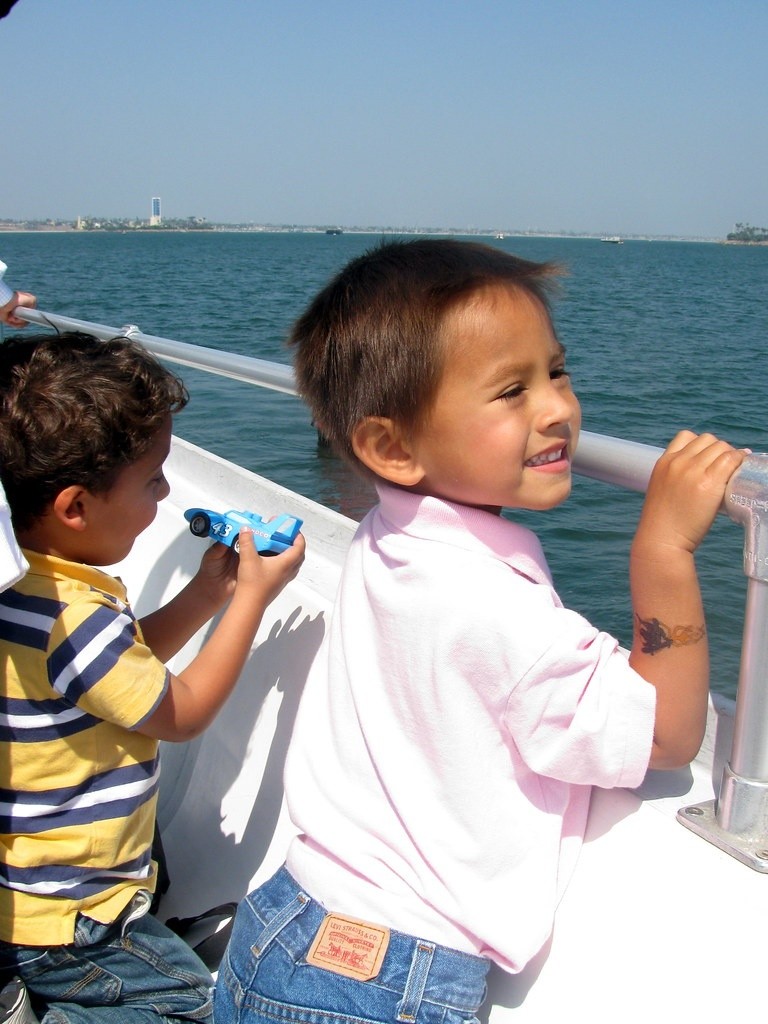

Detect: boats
[601,236,624,245]
[495,233,506,241]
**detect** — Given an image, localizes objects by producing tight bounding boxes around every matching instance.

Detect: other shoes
[0,971,38,1024]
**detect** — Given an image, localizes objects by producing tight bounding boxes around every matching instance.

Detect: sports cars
[182,507,303,559]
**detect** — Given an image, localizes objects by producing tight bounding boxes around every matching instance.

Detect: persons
[0,261,307,1024]
[208,239,752,1024]
